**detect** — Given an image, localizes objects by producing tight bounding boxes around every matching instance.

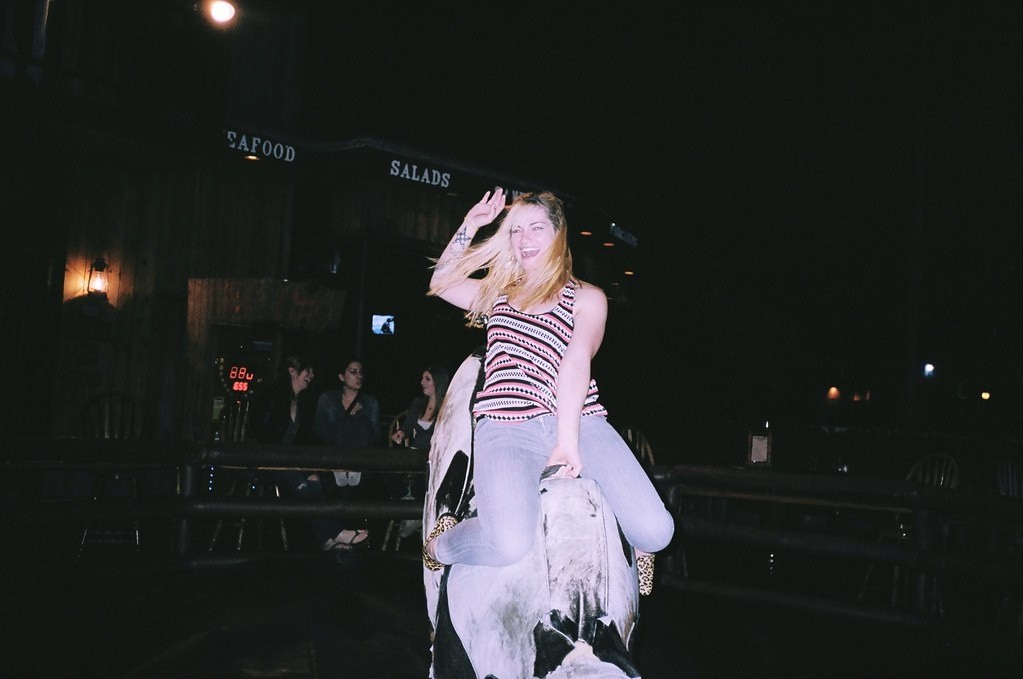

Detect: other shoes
[637,555,655,595]
[422,513,458,571]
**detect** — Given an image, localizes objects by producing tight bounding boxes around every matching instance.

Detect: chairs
[380,409,422,554]
[70,393,159,559]
[623,426,1021,629]
[208,396,290,558]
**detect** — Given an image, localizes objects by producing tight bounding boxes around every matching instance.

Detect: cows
[422,353,640,679]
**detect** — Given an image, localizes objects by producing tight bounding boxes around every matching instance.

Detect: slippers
[324,543,354,556]
[350,528,371,546]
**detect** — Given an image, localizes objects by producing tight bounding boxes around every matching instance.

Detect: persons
[245,352,387,552]
[394,367,449,472]
[421,185,675,598]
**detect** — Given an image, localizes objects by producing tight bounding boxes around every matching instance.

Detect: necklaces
[429,407,431,410]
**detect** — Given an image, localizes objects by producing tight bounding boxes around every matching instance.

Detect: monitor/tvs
[372,314,396,335]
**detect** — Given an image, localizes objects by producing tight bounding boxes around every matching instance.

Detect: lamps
[86,259,109,300]
